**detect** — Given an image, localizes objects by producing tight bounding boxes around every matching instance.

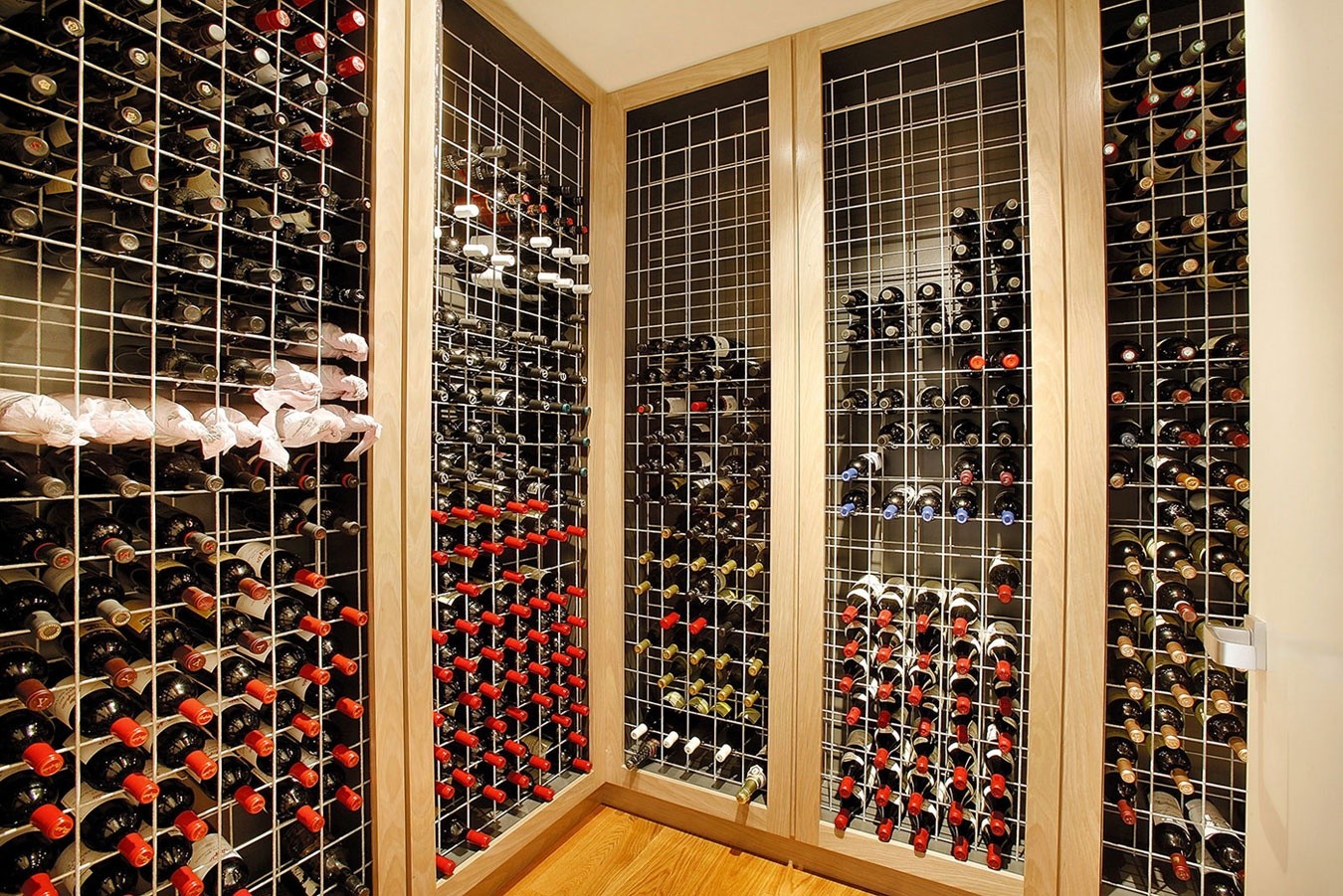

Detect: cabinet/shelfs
[0,0,1252,896]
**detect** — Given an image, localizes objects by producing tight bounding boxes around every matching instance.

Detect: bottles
[0,0,1248,896]
